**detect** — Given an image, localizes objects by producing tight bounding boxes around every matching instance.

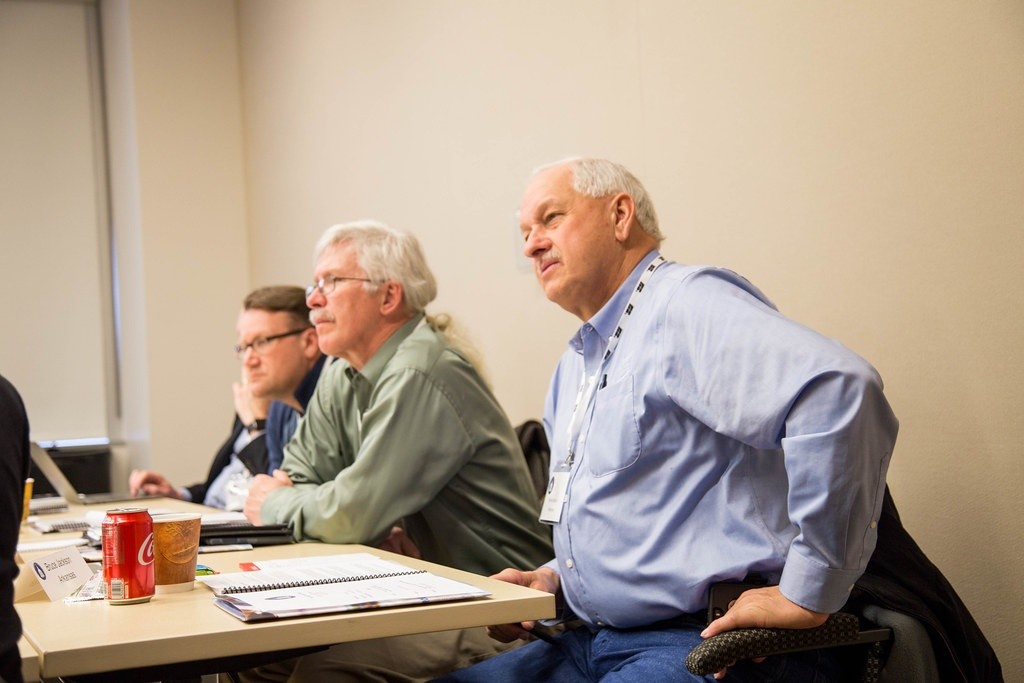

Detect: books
[81,509,296,550]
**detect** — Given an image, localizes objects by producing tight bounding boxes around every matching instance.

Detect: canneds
[102,508,156,605]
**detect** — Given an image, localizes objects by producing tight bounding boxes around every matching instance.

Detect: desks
[13,496,556,683]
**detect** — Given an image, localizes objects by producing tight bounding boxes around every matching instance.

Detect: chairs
[684,481,1006,683]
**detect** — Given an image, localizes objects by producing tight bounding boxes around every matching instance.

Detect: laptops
[29,440,162,506]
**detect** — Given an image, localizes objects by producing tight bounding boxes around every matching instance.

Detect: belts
[671,607,708,629]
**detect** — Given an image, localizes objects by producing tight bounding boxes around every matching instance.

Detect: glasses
[232,328,305,355]
[303,275,372,297]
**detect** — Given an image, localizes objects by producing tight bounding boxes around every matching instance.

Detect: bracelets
[245,419,271,434]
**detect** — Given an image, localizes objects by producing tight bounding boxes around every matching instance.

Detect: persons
[425,158,900,683]
[129,265,330,515]
[0,373,34,683]
[240,220,556,683]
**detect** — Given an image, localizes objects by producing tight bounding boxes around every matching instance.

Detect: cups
[151,513,202,594]
[20,478,35,523]
